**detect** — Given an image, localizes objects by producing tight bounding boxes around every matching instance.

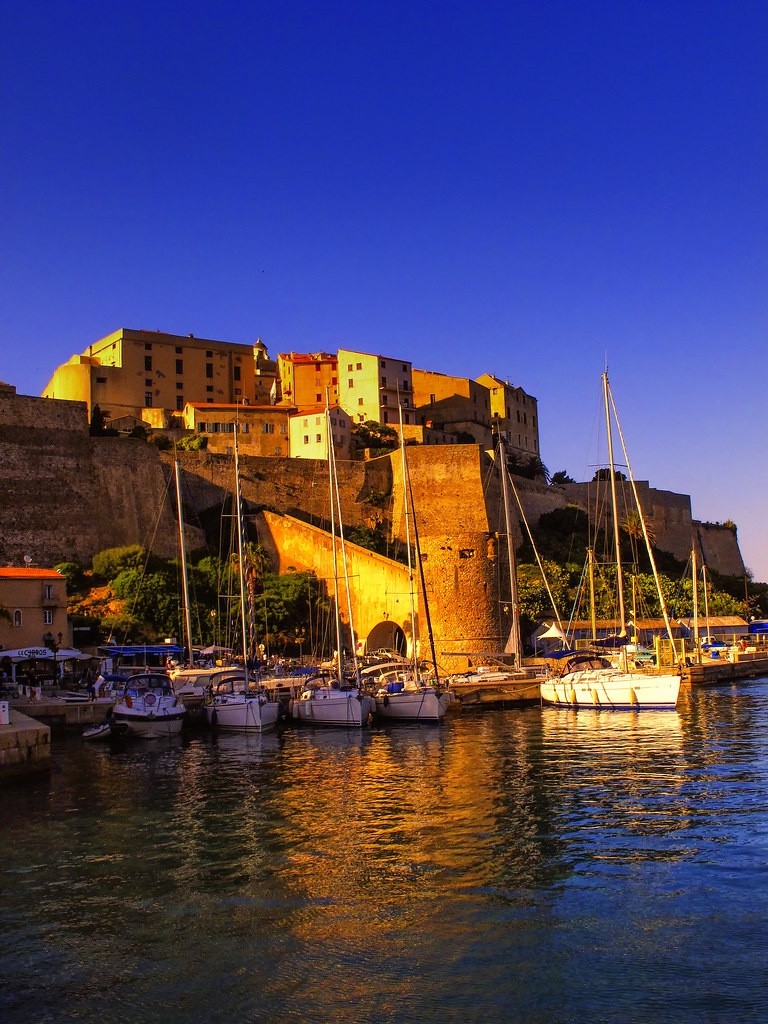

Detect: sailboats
[539,369,684,709]
[120,384,454,737]
[429,416,578,707]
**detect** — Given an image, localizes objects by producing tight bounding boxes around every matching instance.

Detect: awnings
[97,646,184,654]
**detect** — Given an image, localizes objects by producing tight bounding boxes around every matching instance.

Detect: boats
[667,550,768,686]
